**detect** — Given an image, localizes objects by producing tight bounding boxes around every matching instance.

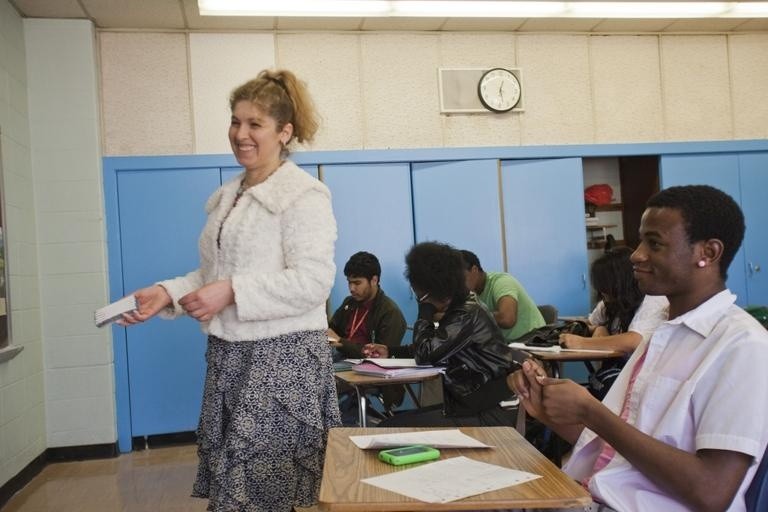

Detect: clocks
[478,68,521,113]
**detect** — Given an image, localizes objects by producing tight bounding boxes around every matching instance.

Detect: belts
[584,502,616,512]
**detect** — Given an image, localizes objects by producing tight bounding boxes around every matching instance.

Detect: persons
[330,251,408,410]
[459,249,547,343]
[559,245,669,396]
[361,242,536,427]
[113,72,337,510]
[506,185,767,510]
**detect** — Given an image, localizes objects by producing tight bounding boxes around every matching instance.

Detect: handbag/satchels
[526,320,590,347]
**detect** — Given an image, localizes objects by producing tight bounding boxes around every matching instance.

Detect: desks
[511,347,623,434]
[335,370,440,427]
[318,425,591,511]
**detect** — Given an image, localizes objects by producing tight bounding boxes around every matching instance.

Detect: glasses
[415,292,431,303]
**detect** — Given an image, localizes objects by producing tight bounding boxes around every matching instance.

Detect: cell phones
[380,446,441,467]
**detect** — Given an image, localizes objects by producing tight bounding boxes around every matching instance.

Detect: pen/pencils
[371,329,375,345]
[511,360,522,367]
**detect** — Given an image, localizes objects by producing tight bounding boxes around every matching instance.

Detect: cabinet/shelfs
[739,151,768,308]
[221,163,321,183]
[410,157,507,280]
[580,154,660,309]
[659,153,748,308]
[498,157,591,321]
[319,162,420,415]
[116,167,221,437]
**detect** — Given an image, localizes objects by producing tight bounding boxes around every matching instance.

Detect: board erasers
[95,295,139,327]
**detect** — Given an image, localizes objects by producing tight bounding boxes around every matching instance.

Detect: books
[332,358,447,379]
